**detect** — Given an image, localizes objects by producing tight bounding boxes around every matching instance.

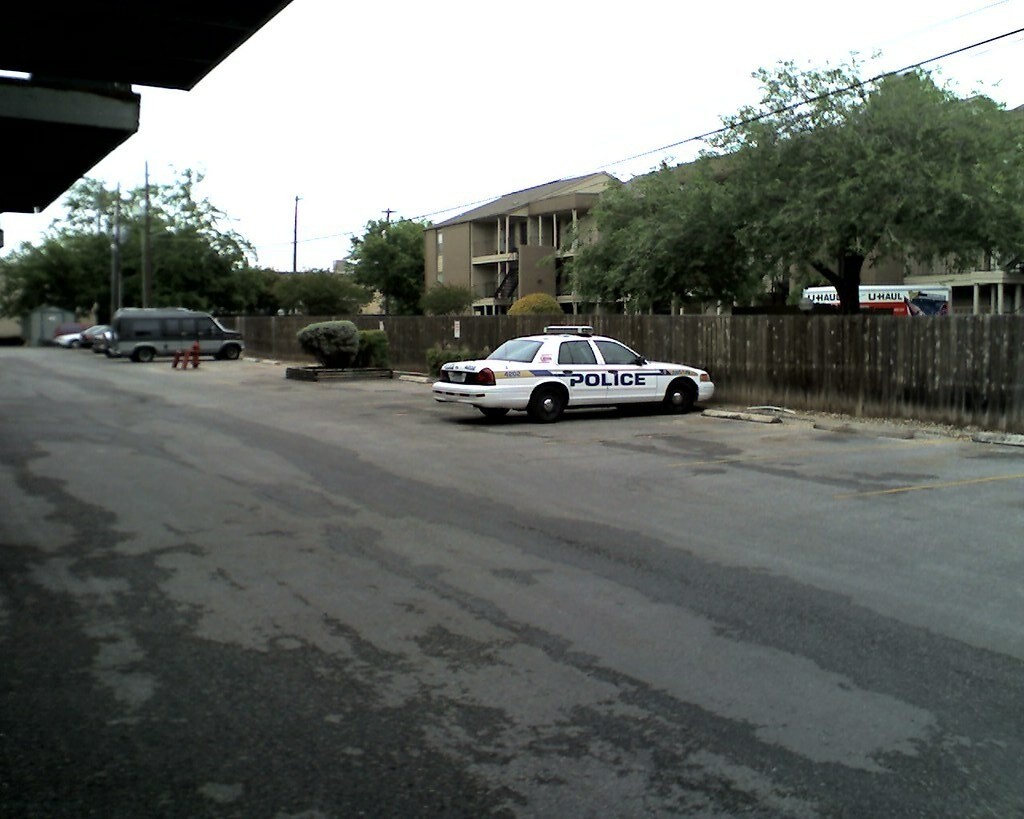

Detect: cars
[432,325,715,425]
[92,328,120,358]
[55,333,84,349]
[80,324,110,349]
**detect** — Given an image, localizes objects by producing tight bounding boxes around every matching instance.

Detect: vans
[110,307,245,362]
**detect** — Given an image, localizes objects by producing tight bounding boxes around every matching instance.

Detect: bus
[802,287,953,317]
